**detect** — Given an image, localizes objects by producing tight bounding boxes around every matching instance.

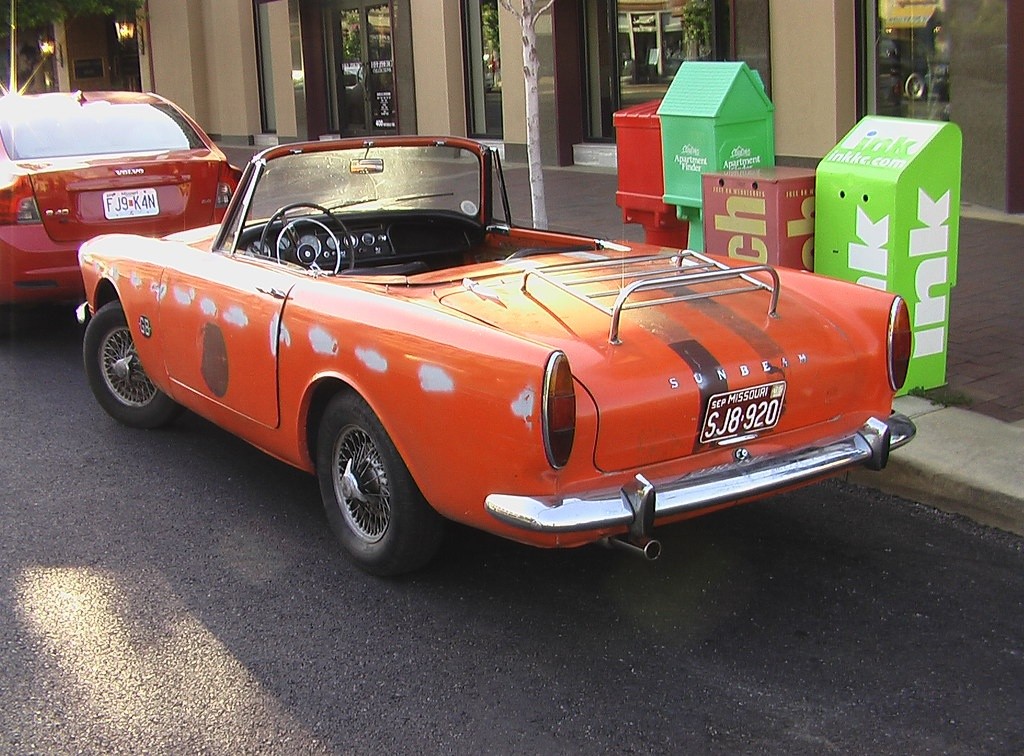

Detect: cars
[0,91,242,335]
[880,35,948,102]
[75,134,919,579]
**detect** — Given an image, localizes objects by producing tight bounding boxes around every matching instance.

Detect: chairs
[338,262,428,276]
[506,244,593,259]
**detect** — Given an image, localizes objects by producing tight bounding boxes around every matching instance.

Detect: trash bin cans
[612,100,691,250]
[699,165,817,272]
[814,114,962,398]
[657,60,775,256]
